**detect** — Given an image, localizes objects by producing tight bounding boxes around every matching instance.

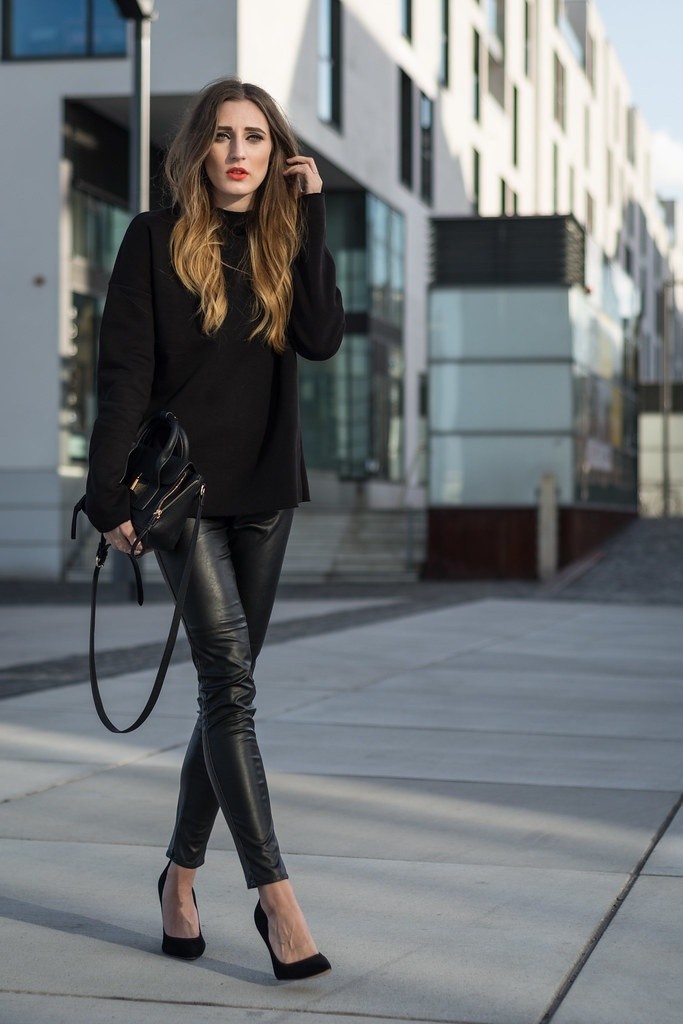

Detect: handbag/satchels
[68,411,207,734]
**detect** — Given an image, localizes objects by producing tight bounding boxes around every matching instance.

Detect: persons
[85,80,349,982]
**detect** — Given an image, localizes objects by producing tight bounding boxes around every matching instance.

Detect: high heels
[253,898,332,980]
[157,860,206,960]
